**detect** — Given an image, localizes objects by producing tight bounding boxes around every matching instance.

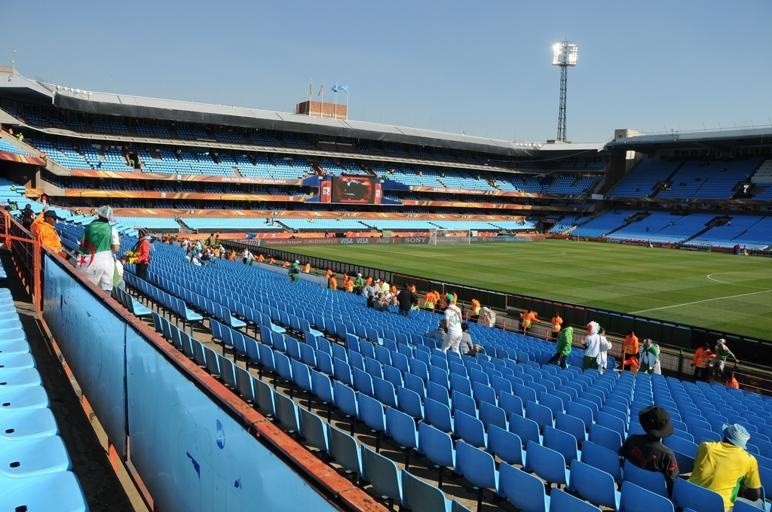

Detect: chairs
[2,206,772,512]
[1,111,604,234]
[569,147,769,254]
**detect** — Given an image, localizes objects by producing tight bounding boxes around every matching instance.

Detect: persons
[326,268,418,317]
[6,198,62,256]
[421,287,474,359]
[488,178,499,189]
[692,339,738,388]
[687,422,762,512]
[116,257,126,293]
[619,407,680,499]
[129,228,150,280]
[733,243,740,255]
[467,296,496,328]
[288,259,301,283]
[549,320,573,367]
[580,321,612,368]
[519,307,538,335]
[162,229,254,266]
[620,332,661,374]
[552,312,563,337]
[74,204,120,296]
[740,244,750,257]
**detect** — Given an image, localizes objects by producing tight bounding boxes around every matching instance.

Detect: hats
[96,205,113,222]
[639,405,673,438]
[445,294,454,302]
[587,321,601,334]
[139,227,147,236]
[44,211,59,219]
[722,423,751,450]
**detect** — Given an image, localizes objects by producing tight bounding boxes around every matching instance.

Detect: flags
[317,82,324,97]
[307,81,313,97]
[329,82,337,96]
[338,83,351,96]
